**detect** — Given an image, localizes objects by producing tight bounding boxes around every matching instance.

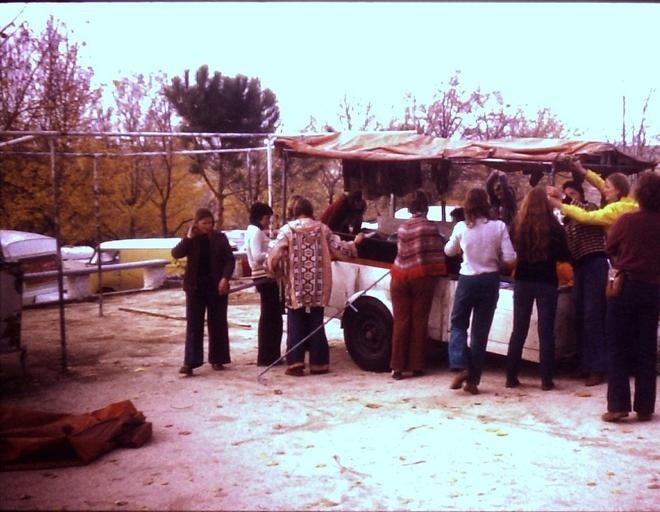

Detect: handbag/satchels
[606,268,623,297]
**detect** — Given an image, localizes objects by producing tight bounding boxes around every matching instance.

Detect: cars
[58,244,96,290]
[219,230,247,253]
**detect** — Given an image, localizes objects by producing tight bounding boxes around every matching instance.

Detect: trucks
[87,236,188,300]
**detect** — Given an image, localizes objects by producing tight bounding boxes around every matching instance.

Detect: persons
[390,193,447,379]
[245,191,368,377]
[171,208,235,374]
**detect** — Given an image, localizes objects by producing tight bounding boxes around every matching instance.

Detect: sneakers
[542,382,554,391]
[285,368,304,376]
[586,374,602,386]
[212,363,224,371]
[506,378,520,387]
[450,369,478,394]
[603,410,629,421]
[391,372,401,380]
[180,366,192,375]
[412,370,425,376]
[637,412,652,421]
